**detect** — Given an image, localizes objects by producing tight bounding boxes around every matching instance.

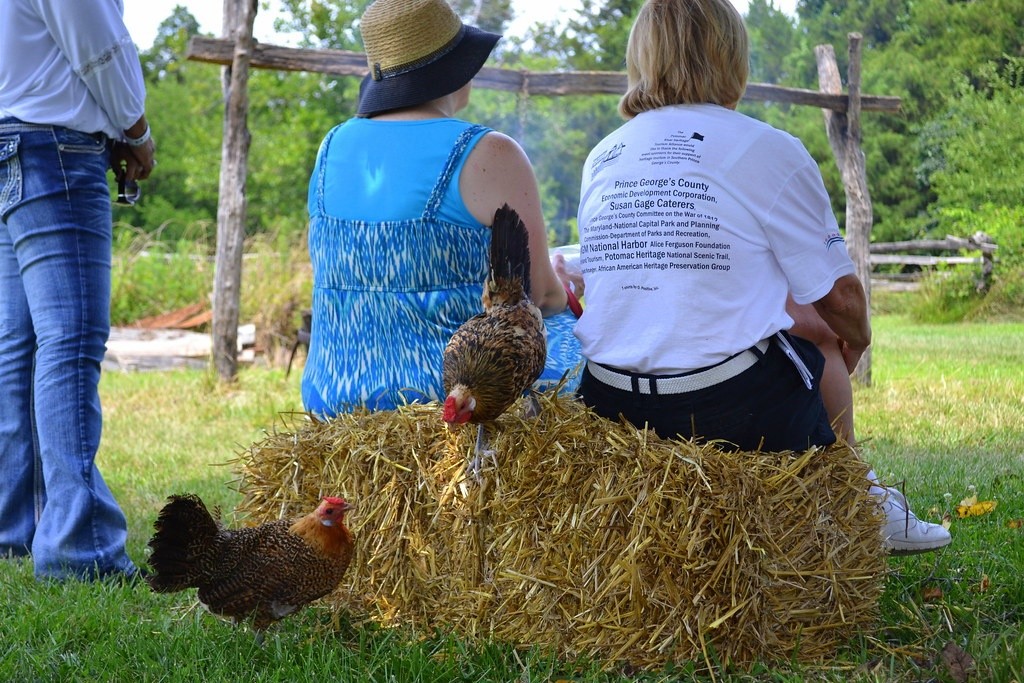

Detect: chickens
[142,491,354,645]
[443,202,545,479]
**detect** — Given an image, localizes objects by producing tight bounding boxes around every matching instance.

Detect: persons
[572,0,952,552]
[0,0,154,587]
[301,0,588,429]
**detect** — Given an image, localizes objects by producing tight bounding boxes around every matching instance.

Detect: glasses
[111,169,141,208]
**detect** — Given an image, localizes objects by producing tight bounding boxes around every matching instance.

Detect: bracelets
[123,124,150,146]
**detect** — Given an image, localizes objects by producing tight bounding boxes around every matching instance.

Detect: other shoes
[879,487,952,551]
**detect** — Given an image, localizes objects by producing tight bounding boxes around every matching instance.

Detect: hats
[357,0,504,116]
